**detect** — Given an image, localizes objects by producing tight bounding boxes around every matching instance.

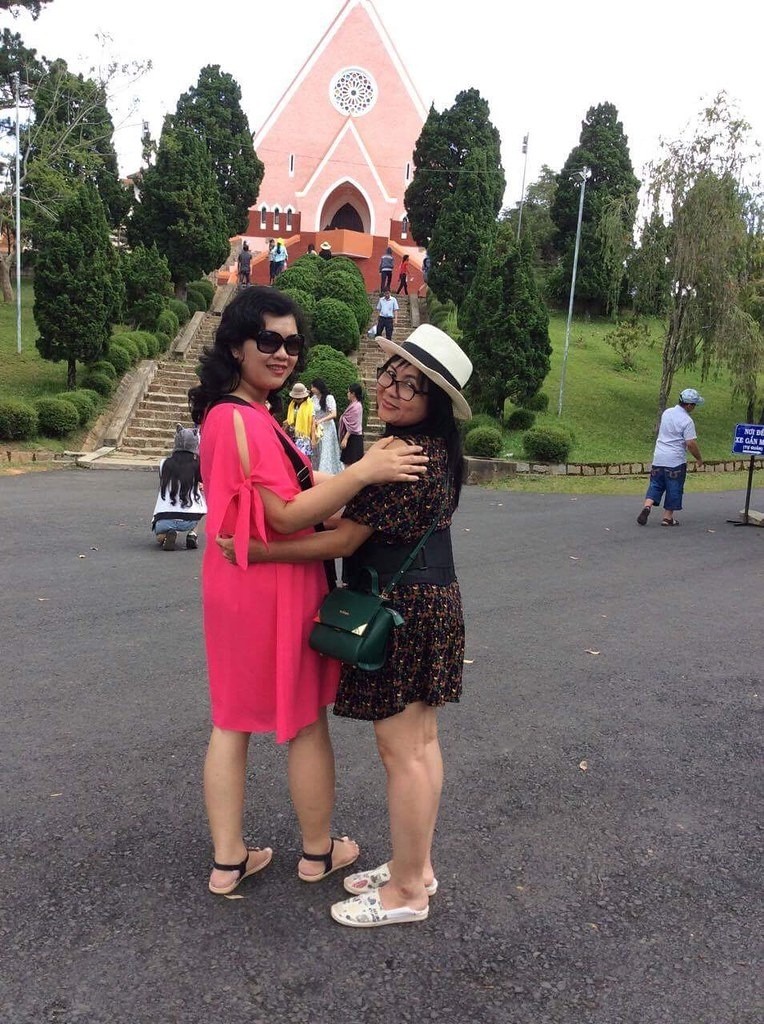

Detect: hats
[375,323,473,421]
[680,388,704,404]
[276,238,284,244]
[288,384,310,398]
[320,241,331,250]
[387,247,392,252]
[172,424,200,454]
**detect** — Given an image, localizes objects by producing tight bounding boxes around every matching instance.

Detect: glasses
[377,367,428,401]
[243,330,306,355]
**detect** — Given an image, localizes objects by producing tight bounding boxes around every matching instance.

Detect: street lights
[557,165,592,418]
[8,70,35,355]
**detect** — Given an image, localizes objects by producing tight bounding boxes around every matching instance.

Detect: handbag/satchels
[310,589,406,671]
[367,325,377,338]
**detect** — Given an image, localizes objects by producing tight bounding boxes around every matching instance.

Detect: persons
[396,255,412,295]
[151,424,208,549]
[319,242,332,260]
[265,379,364,476]
[269,238,288,285]
[238,246,253,288]
[379,248,395,292]
[188,285,429,894]
[637,389,704,526]
[307,244,316,254]
[216,323,473,926]
[418,258,430,296]
[377,288,399,340]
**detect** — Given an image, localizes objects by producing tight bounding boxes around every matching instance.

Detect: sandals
[637,506,650,526]
[208,846,273,893]
[661,517,680,526]
[298,838,359,882]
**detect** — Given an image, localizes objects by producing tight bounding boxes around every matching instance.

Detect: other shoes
[331,890,429,927]
[344,863,438,895]
[186,531,199,549]
[162,531,177,550]
